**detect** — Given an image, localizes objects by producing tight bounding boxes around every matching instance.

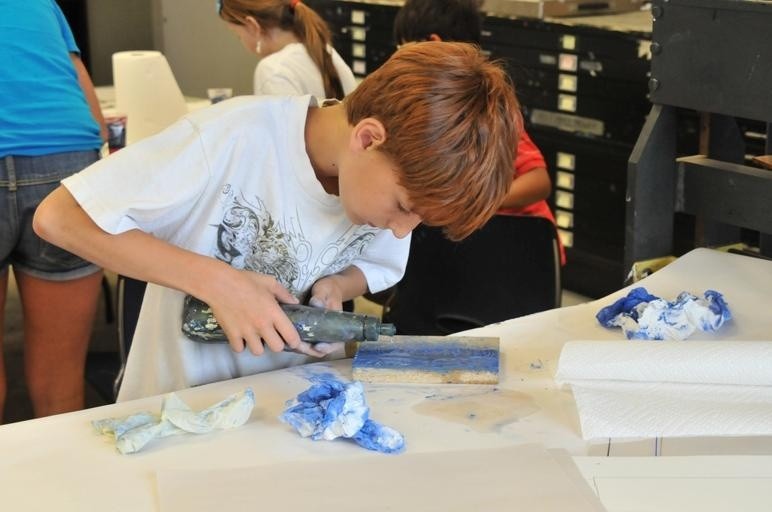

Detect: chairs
[110,271,148,404]
[379,212,563,340]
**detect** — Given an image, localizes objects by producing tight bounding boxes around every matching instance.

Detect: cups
[205,88,232,104]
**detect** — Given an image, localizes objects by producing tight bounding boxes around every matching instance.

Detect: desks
[0,246,771,512]
[95,85,212,163]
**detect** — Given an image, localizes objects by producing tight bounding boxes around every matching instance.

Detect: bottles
[182,295,399,346]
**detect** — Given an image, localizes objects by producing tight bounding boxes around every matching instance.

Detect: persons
[0,0,110,425]
[393,0,567,335]
[218,0,356,313]
[33,41,523,404]
